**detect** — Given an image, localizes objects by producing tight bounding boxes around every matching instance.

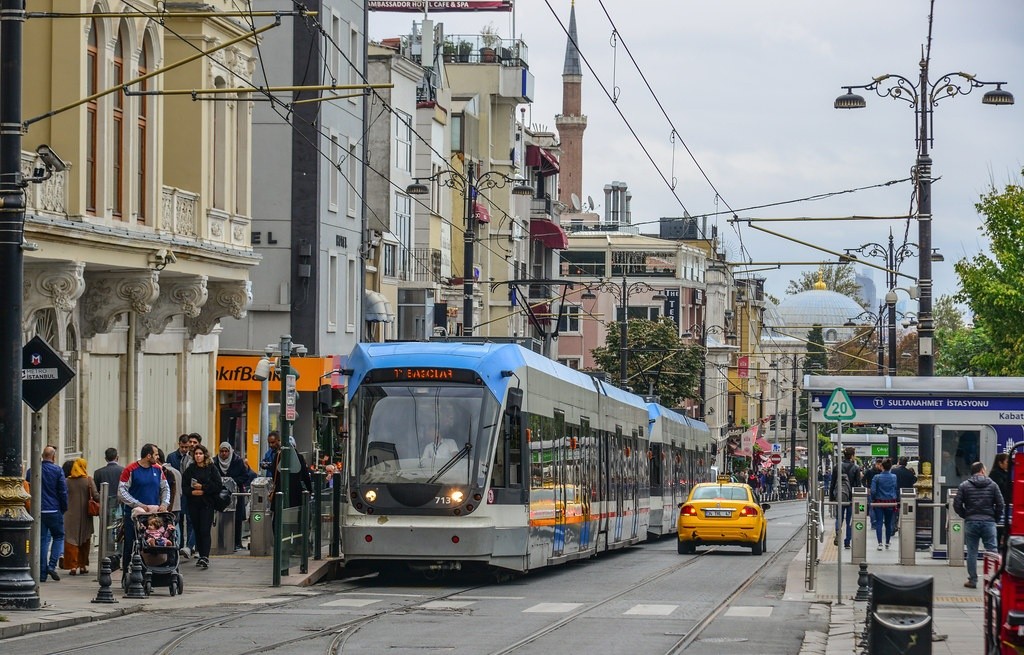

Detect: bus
[316,279,650,582]
[629,348,719,540]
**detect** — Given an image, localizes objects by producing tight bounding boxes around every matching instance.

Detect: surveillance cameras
[254,359,270,381]
[901,318,910,328]
[35,144,66,172]
[155,249,167,259]
[290,343,308,358]
[885,291,897,309]
[166,250,177,263]
[266,344,278,357]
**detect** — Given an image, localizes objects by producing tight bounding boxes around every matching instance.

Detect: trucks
[982,440,1024,655]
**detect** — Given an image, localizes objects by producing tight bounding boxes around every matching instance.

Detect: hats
[289,436,296,449]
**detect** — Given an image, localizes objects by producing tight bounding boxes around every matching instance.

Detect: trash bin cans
[870,573,933,655]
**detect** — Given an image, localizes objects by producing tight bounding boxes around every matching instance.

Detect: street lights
[843,298,917,462]
[405,159,537,336]
[833,44,1016,552]
[681,320,737,422]
[581,275,668,392]
[768,348,822,498]
[839,225,945,465]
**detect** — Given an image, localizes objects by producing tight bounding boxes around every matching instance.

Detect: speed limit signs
[823,387,856,420]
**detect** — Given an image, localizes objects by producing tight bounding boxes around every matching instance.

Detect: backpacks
[833,462,854,502]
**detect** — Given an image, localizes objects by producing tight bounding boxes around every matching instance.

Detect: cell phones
[191,478,197,488]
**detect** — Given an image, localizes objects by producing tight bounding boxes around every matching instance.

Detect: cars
[677,474,772,555]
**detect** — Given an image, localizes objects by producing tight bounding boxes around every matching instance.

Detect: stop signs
[771,455,781,464]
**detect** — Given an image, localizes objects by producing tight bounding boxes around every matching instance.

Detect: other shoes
[878,544,883,550]
[964,581,976,589]
[70,568,76,575]
[196,559,201,566]
[80,567,88,574]
[235,543,245,551]
[845,544,851,549]
[885,544,889,549]
[109,555,121,572]
[199,557,209,567]
[58,556,64,569]
[47,569,60,581]
[834,540,838,546]
[181,546,192,559]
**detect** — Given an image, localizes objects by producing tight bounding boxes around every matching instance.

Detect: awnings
[733,438,772,456]
[472,202,489,225]
[365,288,395,323]
[529,303,551,326]
[530,219,568,250]
[526,144,560,177]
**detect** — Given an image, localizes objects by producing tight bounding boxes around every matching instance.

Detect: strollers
[133,512,184,597]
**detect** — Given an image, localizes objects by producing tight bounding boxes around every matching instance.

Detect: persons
[720,466,805,502]
[870,458,897,550]
[953,462,1004,588]
[326,464,340,488]
[829,446,860,549]
[420,423,460,458]
[861,456,918,536]
[268,436,312,514]
[942,451,955,479]
[259,431,280,482]
[988,453,1012,554]
[26,432,258,582]
[140,515,175,548]
[117,444,171,592]
[818,469,831,496]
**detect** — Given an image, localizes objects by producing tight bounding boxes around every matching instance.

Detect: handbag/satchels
[88,477,100,516]
[268,484,276,502]
